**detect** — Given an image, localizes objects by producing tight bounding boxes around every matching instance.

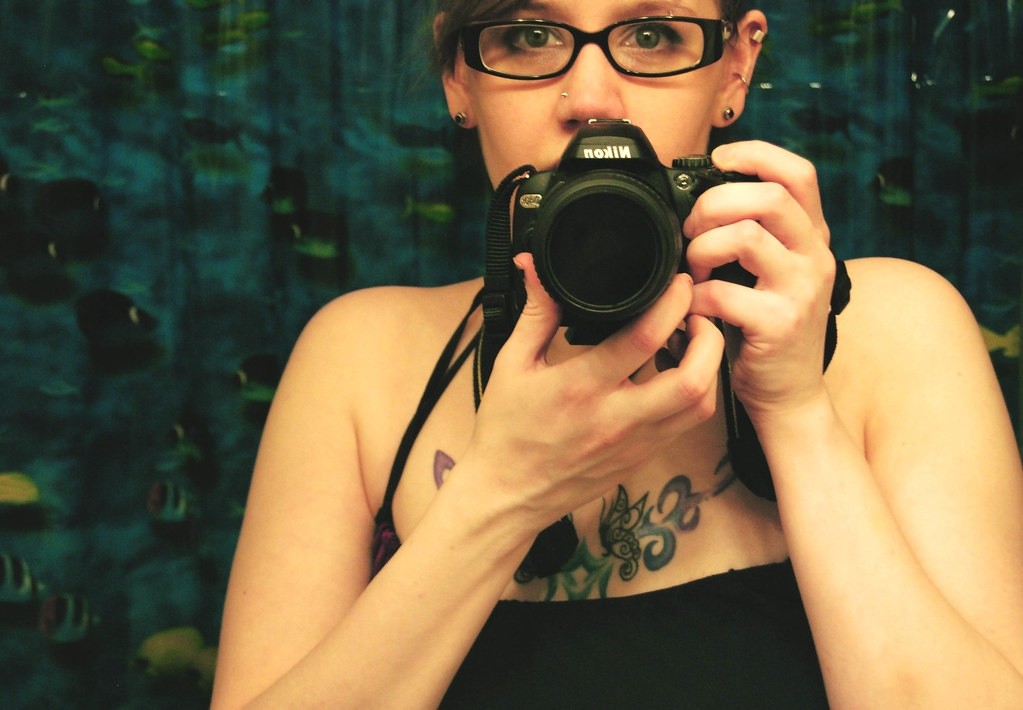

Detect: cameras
[511,118,740,329]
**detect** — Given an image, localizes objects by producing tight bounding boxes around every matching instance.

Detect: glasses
[452,14,739,80]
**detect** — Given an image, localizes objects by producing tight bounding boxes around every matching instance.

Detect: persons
[209,0,1023,710]
